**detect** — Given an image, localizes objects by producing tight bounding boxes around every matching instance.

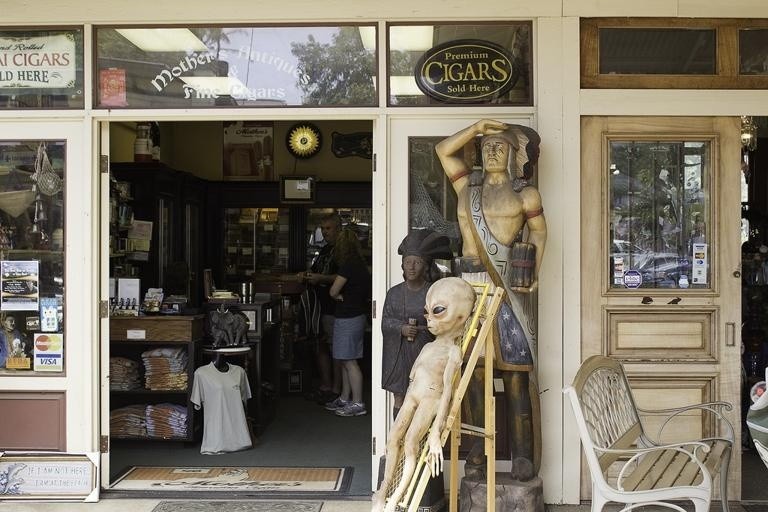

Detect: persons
[324,227,368,419]
[0,315,27,368]
[295,214,346,406]
[379,252,434,436]
[367,276,478,509]
[192,353,255,456]
[432,116,550,483]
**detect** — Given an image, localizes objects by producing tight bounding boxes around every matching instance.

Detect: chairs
[568,355,733,512]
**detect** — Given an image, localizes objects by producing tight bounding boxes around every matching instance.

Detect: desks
[109,313,204,445]
[207,298,281,405]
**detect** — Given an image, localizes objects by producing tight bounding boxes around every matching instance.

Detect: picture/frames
[0,452,101,503]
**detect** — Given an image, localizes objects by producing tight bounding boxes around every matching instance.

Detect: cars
[613,239,692,287]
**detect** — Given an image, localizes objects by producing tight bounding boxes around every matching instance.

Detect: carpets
[150,500,325,512]
[102,466,355,499]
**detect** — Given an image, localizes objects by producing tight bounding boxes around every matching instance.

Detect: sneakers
[305,388,368,417]
